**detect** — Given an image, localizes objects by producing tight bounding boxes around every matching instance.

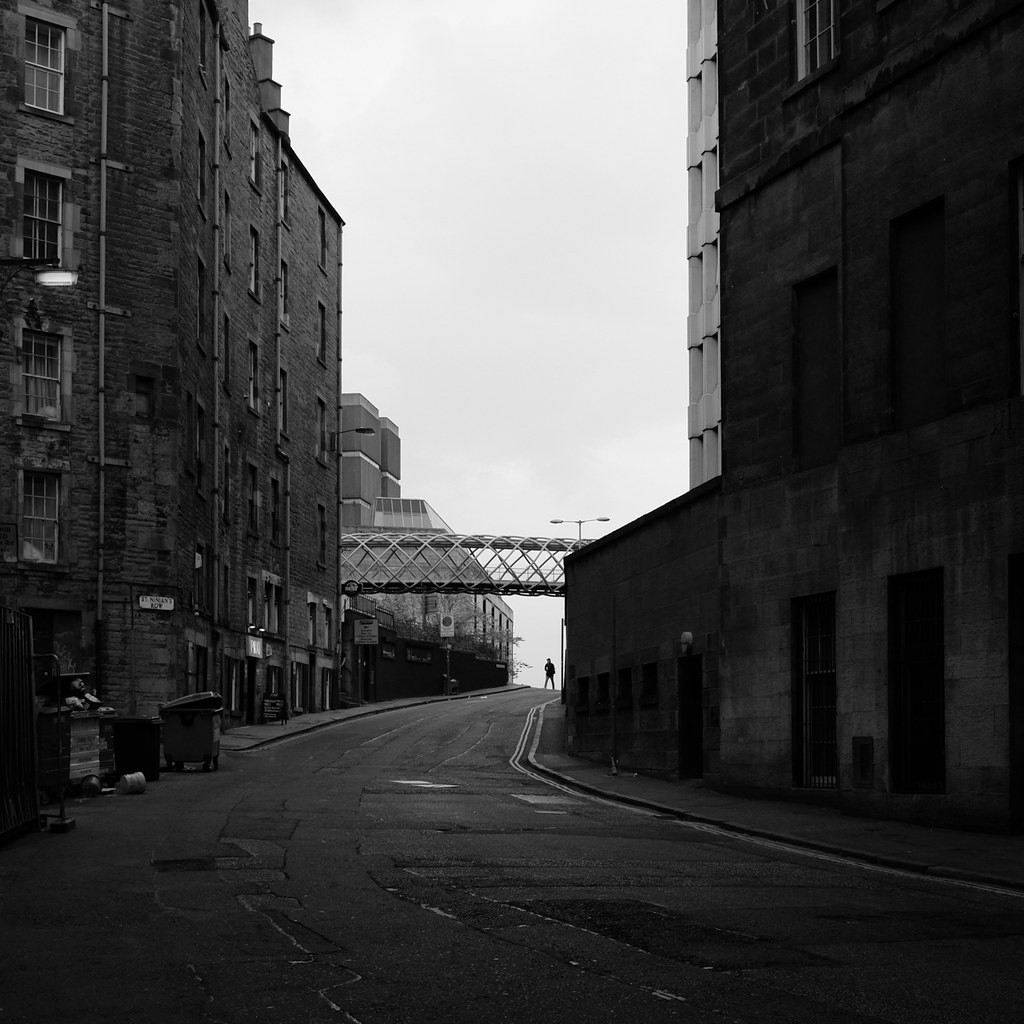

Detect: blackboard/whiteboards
[263,693,285,719]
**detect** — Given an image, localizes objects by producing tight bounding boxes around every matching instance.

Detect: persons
[545,659,555,690]
[279,700,289,725]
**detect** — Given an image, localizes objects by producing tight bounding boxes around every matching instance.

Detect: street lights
[550,516,611,550]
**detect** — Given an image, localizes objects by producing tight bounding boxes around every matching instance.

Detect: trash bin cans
[158,692,226,771]
[35,701,121,807]
[116,714,165,782]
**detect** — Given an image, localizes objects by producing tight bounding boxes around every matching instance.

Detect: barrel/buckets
[80,774,103,797]
[120,772,147,795]
